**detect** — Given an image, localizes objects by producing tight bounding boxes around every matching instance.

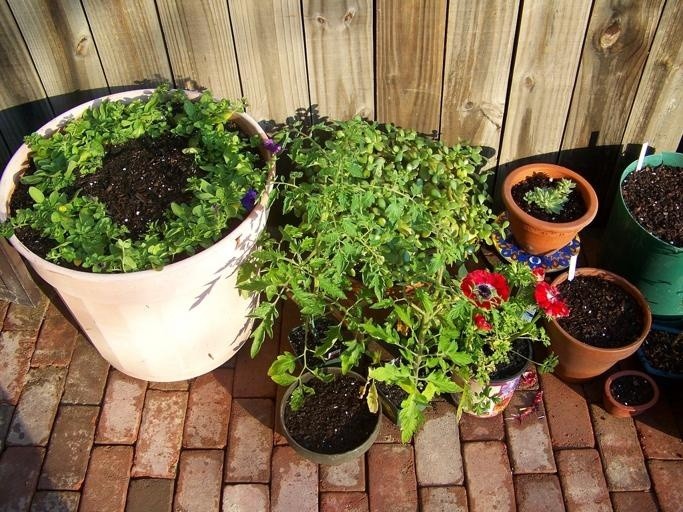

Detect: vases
[278,364,385,468]
[545,267,653,383]
[547,151,681,423]
[604,371,659,420]
[435,268,570,420]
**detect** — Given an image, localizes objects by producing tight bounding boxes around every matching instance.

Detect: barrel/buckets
[603,151,683,318]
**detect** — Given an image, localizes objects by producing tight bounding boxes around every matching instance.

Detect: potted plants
[280,114,487,321]
[287,319,364,364]
[504,163,602,256]
